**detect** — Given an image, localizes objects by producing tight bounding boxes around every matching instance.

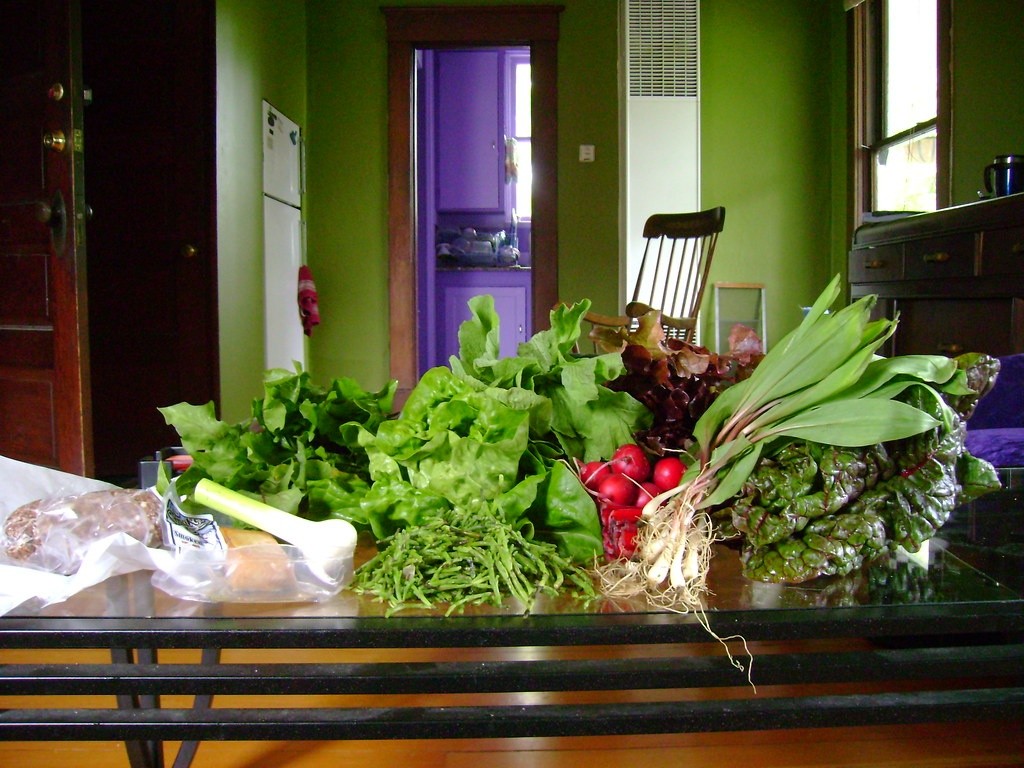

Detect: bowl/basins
[462,227,476,239]
[436,243,450,256]
[451,238,471,254]
[473,241,492,253]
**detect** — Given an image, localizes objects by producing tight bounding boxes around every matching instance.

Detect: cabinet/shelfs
[435,270,533,375]
[437,49,508,215]
[849,190,1024,363]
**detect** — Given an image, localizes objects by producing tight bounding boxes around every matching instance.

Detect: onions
[297,517,358,572]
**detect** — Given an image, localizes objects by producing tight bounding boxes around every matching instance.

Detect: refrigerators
[263,99,301,373]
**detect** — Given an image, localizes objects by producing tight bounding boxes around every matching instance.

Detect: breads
[3,488,160,568]
[220,525,296,590]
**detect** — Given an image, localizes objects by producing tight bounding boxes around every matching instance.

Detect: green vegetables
[152,272,1004,617]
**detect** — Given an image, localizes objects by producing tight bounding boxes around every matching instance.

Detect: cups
[984,154,1024,198]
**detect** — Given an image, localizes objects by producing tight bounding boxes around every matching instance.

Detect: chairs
[551,205,726,362]
